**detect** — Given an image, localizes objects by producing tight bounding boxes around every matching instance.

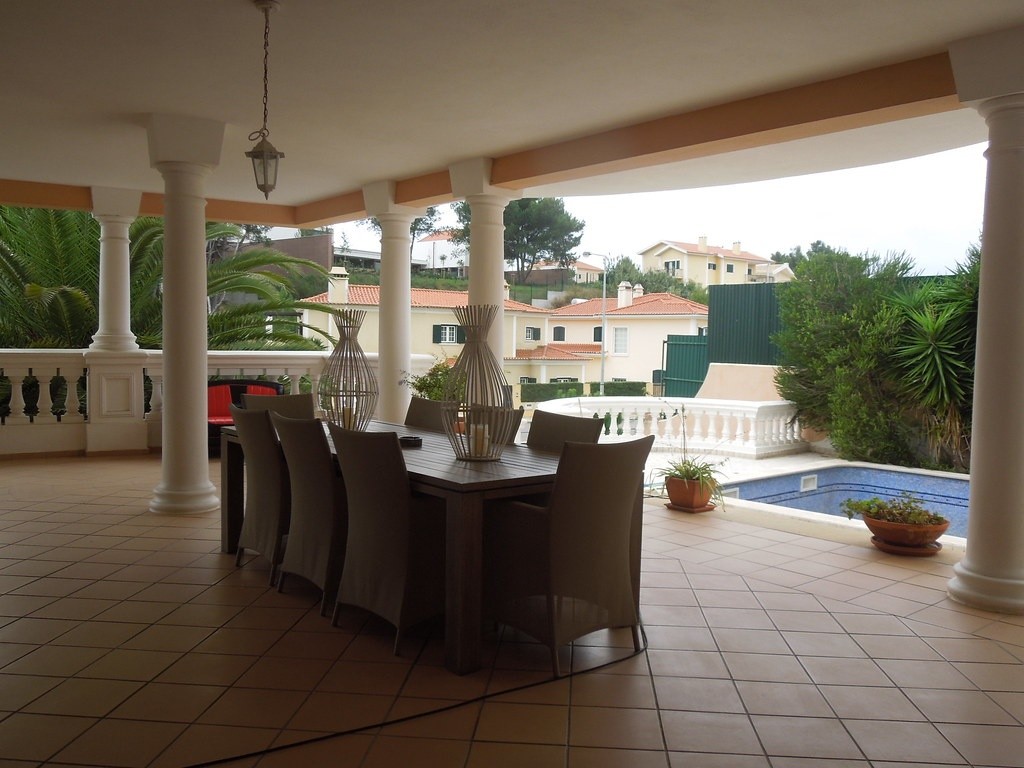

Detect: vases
[663,476,718,513]
[862,515,950,557]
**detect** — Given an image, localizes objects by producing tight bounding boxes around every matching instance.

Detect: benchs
[208,378,284,459]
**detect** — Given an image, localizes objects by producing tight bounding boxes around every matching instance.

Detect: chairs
[463,403,524,445]
[328,421,488,656]
[403,396,461,433]
[240,392,315,426]
[526,409,604,452]
[269,409,349,616]
[229,401,292,587]
[484,435,655,676]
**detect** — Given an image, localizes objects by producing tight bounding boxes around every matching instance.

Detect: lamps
[246,0,284,201]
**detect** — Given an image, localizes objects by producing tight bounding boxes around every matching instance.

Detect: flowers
[641,386,750,514]
[838,491,945,524]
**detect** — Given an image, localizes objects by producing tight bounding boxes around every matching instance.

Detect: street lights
[582,251,608,394]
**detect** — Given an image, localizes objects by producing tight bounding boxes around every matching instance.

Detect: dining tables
[220,418,643,677]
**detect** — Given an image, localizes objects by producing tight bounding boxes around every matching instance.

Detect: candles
[343,406,353,430]
[469,422,489,458]
